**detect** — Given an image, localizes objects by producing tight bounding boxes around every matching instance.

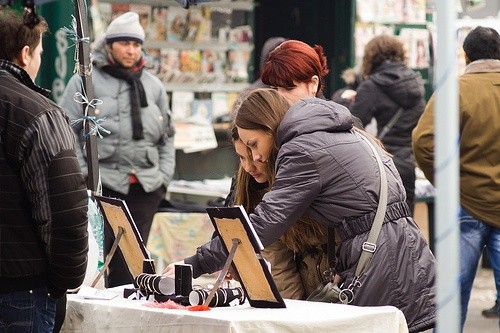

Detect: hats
[106,12,145,45]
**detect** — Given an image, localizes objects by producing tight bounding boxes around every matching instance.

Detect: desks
[146,213,215,275]
[412,197,434,249]
[59,284,409,333]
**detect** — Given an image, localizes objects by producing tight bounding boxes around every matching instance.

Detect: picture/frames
[203,207,287,308]
[91,195,154,289]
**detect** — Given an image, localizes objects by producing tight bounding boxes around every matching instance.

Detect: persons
[0,3,89,333]
[413,26,500,333]
[263,40,328,106]
[162,86,438,333]
[230,127,329,301]
[58,11,176,288]
[348,35,427,219]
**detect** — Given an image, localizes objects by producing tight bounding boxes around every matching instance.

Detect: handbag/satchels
[307,277,348,306]
